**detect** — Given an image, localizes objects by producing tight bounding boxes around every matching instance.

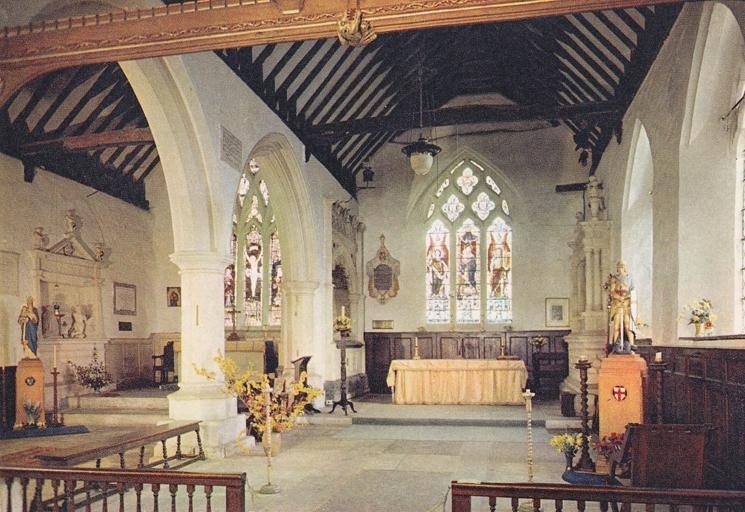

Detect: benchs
[34,417,205,512]
[452,479,744,512]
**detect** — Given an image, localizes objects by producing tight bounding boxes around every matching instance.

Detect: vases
[95,388,100,396]
[536,346,540,353]
[565,454,573,472]
[262,431,281,498]
[596,455,609,474]
[694,323,700,336]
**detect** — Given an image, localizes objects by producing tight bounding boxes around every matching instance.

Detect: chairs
[610,423,708,487]
[152,341,175,384]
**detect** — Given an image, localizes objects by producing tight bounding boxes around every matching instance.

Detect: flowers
[527,337,547,347]
[66,346,115,388]
[677,295,717,325]
[191,348,326,433]
[548,433,583,455]
[589,431,624,456]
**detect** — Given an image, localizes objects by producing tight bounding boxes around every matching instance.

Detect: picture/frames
[113,281,137,316]
[545,298,569,327]
[167,287,181,308]
[270,235,282,310]
[243,233,262,303]
[224,264,235,307]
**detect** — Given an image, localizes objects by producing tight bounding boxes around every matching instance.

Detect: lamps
[400,29,442,175]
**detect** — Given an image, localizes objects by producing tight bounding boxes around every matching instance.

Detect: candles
[415,336,418,346]
[264,378,270,389]
[54,343,56,368]
[526,389,530,395]
[655,352,661,361]
[580,355,587,362]
[502,337,503,346]
[341,305,345,317]
